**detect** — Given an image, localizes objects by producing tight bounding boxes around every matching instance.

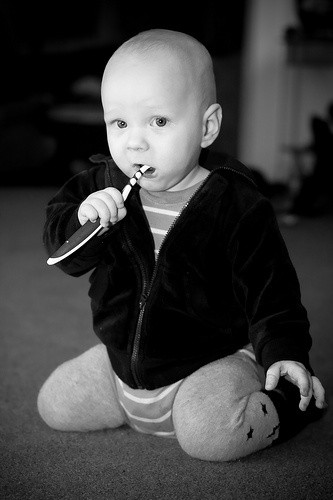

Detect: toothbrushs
[45,164,151,267]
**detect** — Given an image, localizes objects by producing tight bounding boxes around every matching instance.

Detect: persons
[37,29,328,462]
